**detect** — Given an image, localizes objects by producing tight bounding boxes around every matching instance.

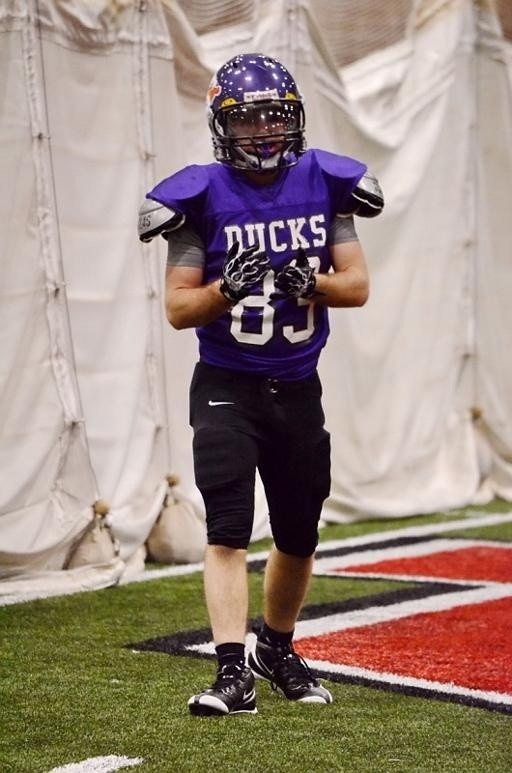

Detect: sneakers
[248,623,333,704]
[187,662,258,715]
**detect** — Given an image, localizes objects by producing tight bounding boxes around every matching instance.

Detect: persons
[132,49,390,720]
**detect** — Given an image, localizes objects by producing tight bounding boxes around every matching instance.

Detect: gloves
[220,242,269,304]
[269,247,315,300]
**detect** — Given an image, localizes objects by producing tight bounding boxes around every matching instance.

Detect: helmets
[205,53,305,173]
